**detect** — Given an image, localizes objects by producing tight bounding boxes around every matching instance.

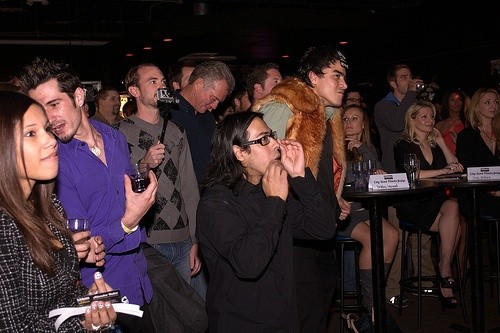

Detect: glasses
[348,98,363,103]
[105,96,124,101]
[243,131,278,146]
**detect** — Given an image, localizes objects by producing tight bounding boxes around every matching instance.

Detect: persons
[194,112,335,333]
[0,45,500,333]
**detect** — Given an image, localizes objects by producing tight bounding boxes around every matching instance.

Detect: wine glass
[403,154,416,189]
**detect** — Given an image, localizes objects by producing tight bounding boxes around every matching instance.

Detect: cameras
[157,88,179,103]
[417,82,424,90]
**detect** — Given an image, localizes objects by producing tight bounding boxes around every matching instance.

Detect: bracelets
[121,221,138,235]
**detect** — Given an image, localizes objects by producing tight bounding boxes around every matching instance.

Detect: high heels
[439,282,458,312]
[435,262,456,288]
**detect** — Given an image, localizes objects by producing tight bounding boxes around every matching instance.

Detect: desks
[338,173,500,333]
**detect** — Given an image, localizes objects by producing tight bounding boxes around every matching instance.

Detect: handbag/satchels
[346,305,402,333]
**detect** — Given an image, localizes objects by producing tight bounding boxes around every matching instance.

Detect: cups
[412,160,421,184]
[353,159,374,183]
[66,219,88,245]
[354,177,373,193]
[126,164,149,192]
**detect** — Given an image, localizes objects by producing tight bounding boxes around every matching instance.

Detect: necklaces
[85,122,101,156]
[478,124,494,145]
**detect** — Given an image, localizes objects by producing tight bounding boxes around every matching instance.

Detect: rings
[91,324,101,331]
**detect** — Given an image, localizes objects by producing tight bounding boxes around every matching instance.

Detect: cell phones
[77,289,120,306]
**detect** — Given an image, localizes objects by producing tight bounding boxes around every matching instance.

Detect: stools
[328,234,370,333]
[397,221,467,329]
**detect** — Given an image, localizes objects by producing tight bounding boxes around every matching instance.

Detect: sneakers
[387,294,409,308]
